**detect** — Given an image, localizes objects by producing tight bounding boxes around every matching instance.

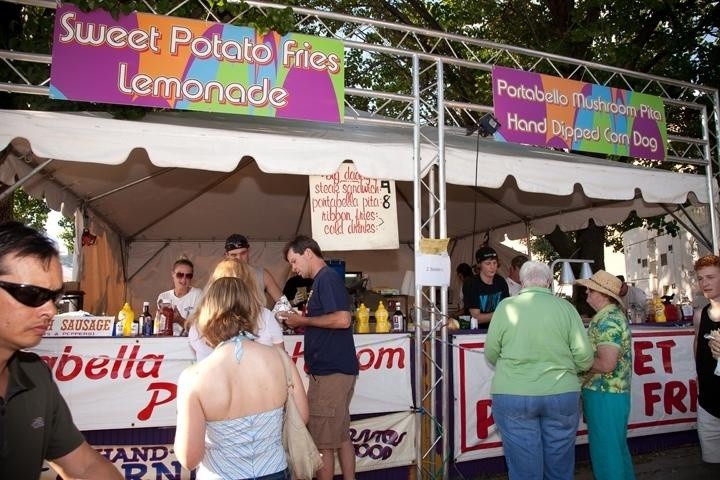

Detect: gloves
[293,290,305,305]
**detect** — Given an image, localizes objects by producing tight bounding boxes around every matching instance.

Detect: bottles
[627,308,633,325]
[653,296,668,323]
[373,301,390,333]
[355,303,371,333]
[680,296,694,321]
[636,311,642,324]
[121,301,135,336]
[138,302,151,337]
[391,302,404,333]
[158,298,174,337]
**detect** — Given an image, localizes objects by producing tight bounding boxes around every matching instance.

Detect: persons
[0,221,125,480]
[575,269,636,479]
[225,234,283,309]
[174,276,310,480]
[157,253,202,336]
[283,236,359,480]
[484,259,593,479]
[187,259,285,362]
[281,274,315,311]
[693,256,720,479]
[616,275,647,325]
[456,247,528,329]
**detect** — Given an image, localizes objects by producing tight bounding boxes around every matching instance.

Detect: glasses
[173,270,195,279]
[1,281,67,307]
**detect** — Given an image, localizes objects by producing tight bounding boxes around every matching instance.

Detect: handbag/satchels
[281,389,324,479]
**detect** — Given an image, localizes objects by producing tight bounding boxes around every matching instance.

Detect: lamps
[460,108,501,137]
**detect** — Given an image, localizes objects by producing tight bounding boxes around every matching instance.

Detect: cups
[648,313,655,322]
[459,315,471,329]
[470,318,479,331]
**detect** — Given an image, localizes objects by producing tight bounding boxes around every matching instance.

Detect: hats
[575,269,626,310]
[475,246,499,264]
[225,234,251,252]
